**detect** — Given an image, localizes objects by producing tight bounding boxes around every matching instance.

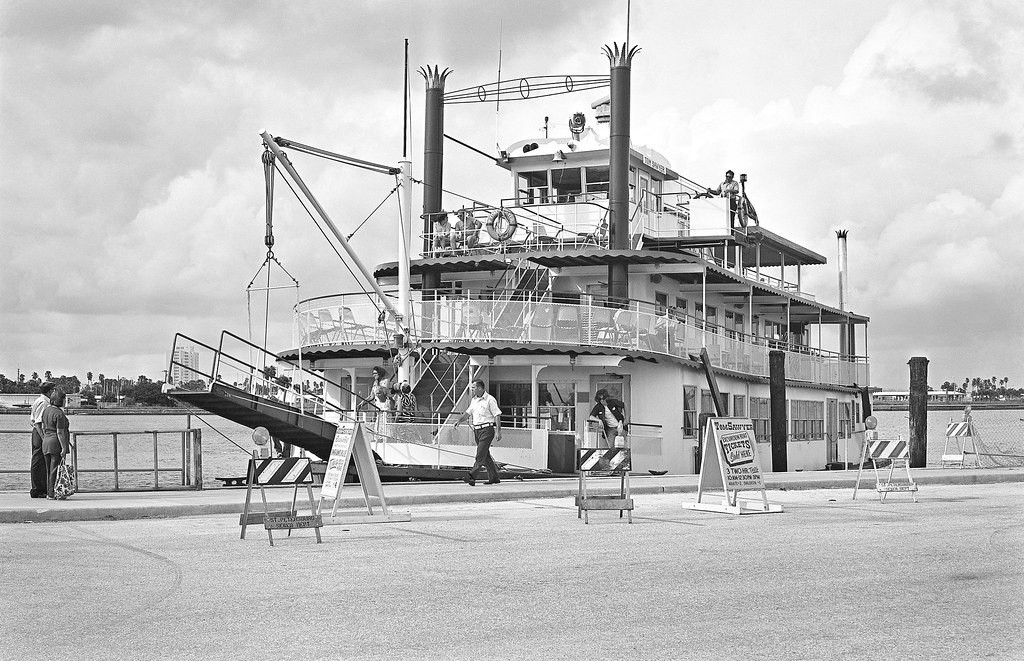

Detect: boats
[161,2,885,483]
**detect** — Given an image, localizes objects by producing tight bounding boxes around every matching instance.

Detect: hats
[39,381,56,388]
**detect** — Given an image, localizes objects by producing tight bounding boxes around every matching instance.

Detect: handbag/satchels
[54,458,76,498]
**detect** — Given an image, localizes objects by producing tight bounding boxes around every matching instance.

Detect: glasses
[726,176,732,178]
[599,397,605,400]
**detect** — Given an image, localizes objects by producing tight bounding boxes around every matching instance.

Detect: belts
[474,422,494,430]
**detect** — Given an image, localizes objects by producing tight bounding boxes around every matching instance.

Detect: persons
[710,170,739,236]
[30,381,56,498]
[654,306,678,344]
[589,388,630,447]
[272,376,297,458]
[453,379,502,486]
[357,366,417,422]
[450,209,482,256]
[42,389,70,500]
[434,211,451,258]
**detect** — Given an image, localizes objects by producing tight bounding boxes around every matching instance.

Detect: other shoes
[463,475,475,486]
[483,478,500,484]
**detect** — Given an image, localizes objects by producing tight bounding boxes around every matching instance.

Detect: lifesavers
[486,207,517,241]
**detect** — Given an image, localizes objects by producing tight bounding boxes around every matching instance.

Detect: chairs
[589,308,763,376]
[430,232,481,259]
[458,302,581,343]
[298,307,398,348]
[532,223,558,251]
[579,220,608,251]
